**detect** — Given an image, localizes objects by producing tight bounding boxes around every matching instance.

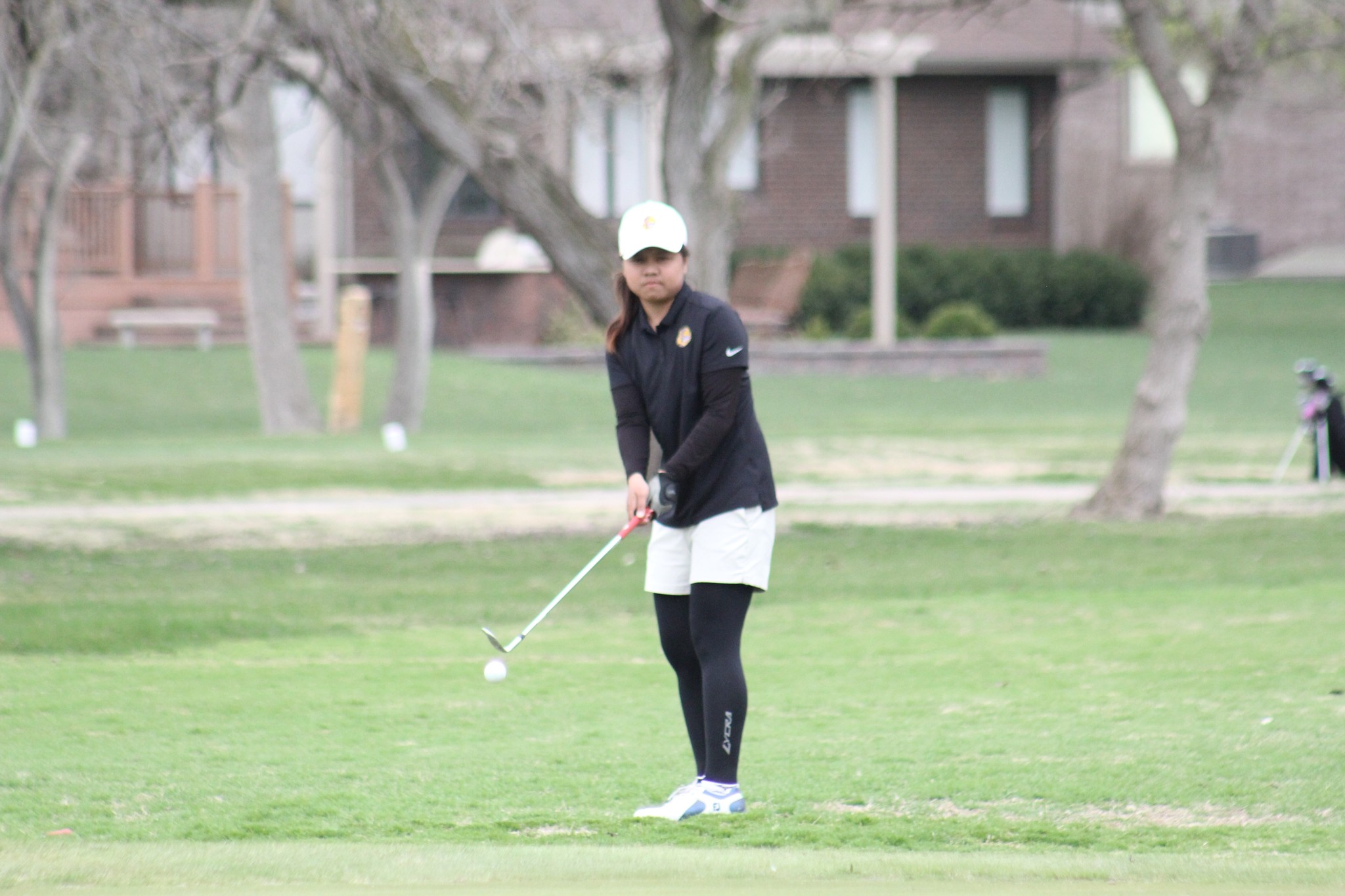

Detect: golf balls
[482,659,504,681]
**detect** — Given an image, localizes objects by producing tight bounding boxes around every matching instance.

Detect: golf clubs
[481,509,654,654]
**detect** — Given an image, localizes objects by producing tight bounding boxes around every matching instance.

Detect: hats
[618,200,688,261]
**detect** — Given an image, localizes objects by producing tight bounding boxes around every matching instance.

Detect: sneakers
[633,775,745,821]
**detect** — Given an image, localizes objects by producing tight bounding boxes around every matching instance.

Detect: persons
[605,201,780,820]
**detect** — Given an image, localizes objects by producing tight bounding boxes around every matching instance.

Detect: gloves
[635,472,679,522]
